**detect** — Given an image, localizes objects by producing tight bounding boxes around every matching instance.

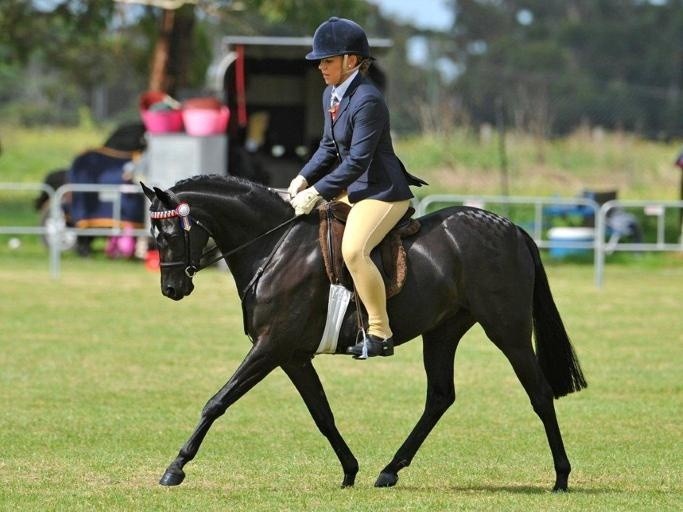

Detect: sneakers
[347,335,395,358]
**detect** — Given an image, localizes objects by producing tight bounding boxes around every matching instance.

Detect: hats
[304,13,378,65]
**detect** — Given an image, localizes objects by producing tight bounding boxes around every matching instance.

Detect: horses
[139,173,589,493]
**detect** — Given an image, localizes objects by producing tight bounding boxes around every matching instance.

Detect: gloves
[282,175,308,199]
[289,187,321,218]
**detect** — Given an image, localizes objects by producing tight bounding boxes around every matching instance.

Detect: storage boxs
[546,226,596,258]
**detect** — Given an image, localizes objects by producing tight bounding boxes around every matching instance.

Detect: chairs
[544,189,618,233]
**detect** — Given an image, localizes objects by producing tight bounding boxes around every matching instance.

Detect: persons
[284,16,431,358]
